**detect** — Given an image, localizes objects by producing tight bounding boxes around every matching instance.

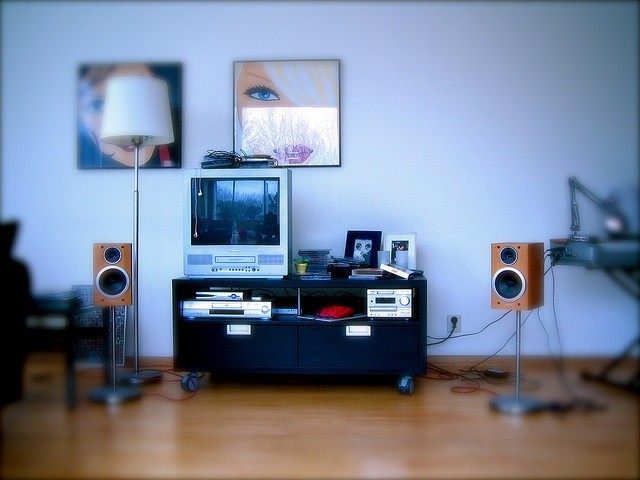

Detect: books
[380,263,415,279]
[330,257,359,268]
[348,275,383,280]
[352,268,388,277]
[294,248,332,280]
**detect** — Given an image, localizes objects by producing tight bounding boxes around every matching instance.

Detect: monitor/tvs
[182,166,292,280]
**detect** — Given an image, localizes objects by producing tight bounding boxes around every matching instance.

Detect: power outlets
[447,315,461,332]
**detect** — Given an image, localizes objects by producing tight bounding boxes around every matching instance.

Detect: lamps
[98,75,175,386]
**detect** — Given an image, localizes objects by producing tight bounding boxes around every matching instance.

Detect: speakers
[92,242,133,307]
[490,240,545,310]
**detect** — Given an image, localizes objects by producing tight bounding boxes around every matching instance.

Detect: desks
[584,263,638,400]
[25,292,111,410]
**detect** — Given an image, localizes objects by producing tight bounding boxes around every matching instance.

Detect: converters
[484,367,510,379]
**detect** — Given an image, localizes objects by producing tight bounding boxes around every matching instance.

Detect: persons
[235,61,338,166]
[352,242,365,262]
[78,63,181,167]
[392,243,406,263]
[360,244,371,265]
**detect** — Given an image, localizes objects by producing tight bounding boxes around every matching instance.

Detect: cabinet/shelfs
[172,265,428,394]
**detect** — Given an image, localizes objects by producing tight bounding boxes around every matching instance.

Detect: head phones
[180,370,204,392]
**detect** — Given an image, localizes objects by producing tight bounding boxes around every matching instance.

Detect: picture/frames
[384,233,417,270]
[344,229,383,268]
